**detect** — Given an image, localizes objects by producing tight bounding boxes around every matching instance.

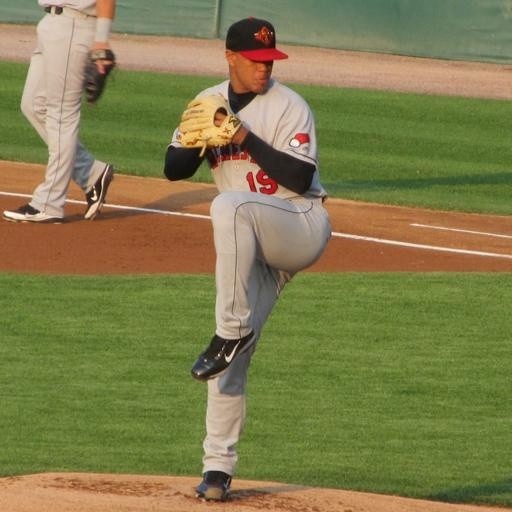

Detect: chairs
[2,203,65,224]
[191,329,257,382]
[196,470,233,503]
[83,163,114,220]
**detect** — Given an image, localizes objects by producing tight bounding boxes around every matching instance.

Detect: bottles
[226,17,288,62]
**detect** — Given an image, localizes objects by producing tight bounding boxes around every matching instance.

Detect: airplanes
[83,49,117,103]
[175,91,242,149]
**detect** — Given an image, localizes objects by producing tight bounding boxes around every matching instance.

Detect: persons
[165,16,331,501]
[2,0,117,223]
